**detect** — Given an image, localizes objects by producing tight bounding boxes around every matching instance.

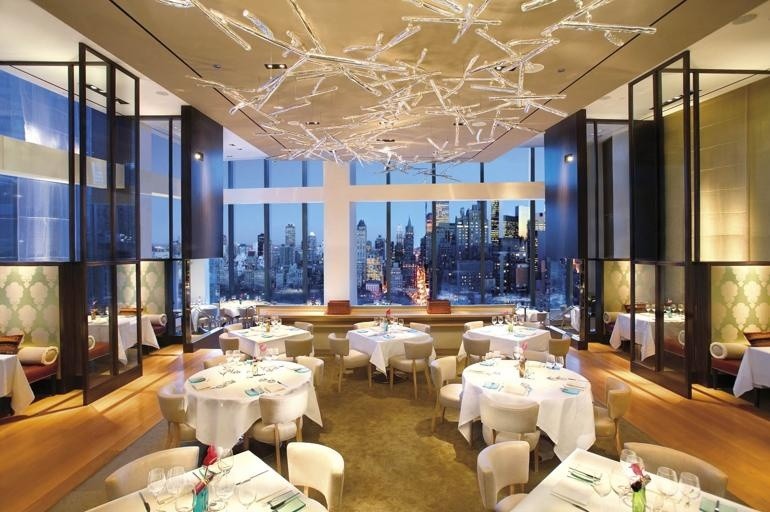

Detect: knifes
[271,492,300,509]
[139,491,150,512]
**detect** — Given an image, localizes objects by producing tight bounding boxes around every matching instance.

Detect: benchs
[711,357,751,390]
[88,336,113,375]
[17,365,58,396]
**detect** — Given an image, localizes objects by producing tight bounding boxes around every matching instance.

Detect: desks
[504,446,766,512]
[86,449,329,512]
[733,346,770,408]
[0,354,35,417]
[87,314,160,368]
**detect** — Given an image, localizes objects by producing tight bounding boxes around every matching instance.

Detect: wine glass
[593,449,701,512]
[255,316,282,333]
[664,303,684,316]
[645,304,655,315]
[492,314,524,327]
[147,446,256,512]
[486,347,568,387]
[218,348,279,383]
[374,316,404,329]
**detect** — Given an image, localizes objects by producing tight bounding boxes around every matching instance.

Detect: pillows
[0,335,23,355]
[709,341,751,359]
[88,336,96,350]
[743,332,770,347]
[15,345,58,367]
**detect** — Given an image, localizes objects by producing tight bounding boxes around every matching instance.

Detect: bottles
[239,297,242,305]
